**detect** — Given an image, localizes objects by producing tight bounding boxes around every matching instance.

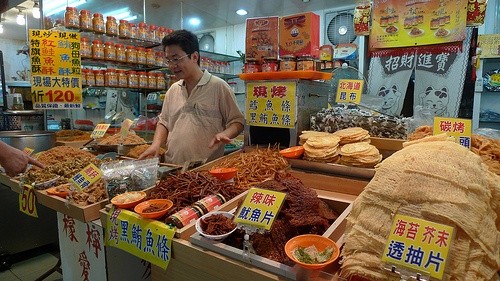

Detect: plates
[384,25,450,38]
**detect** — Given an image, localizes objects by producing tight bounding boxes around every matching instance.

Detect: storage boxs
[104,184,248,238]
[279,11,321,58]
[8,176,37,197]
[319,43,357,61]
[244,17,279,60]
[37,183,109,222]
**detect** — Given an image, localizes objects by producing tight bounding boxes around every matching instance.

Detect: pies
[299,127,383,167]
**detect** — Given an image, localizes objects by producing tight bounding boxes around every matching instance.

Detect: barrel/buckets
[0,130,57,173]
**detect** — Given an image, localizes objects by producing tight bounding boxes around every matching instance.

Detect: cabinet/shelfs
[472,54,500,137]
[39,5,240,137]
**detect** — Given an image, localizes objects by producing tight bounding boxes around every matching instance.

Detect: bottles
[381,13,450,29]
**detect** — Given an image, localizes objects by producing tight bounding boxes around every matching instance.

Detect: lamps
[42,0,93,18]
[98,7,141,26]
[0,0,41,34]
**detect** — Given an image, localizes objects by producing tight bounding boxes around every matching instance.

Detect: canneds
[164,194,226,229]
[200,56,230,75]
[64,7,175,90]
[242,54,346,73]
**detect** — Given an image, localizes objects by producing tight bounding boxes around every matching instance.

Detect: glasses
[164,53,191,66]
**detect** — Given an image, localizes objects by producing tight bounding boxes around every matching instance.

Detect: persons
[137,30,245,165]
[0,140,45,177]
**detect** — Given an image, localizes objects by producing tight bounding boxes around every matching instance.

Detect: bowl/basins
[47,187,69,198]
[208,168,237,180]
[111,191,145,208]
[196,212,238,239]
[92,142,153,155]
[134,198,173,219]
[35,176,60,189]
[278,145,304,157]
[285,234,340,268]
